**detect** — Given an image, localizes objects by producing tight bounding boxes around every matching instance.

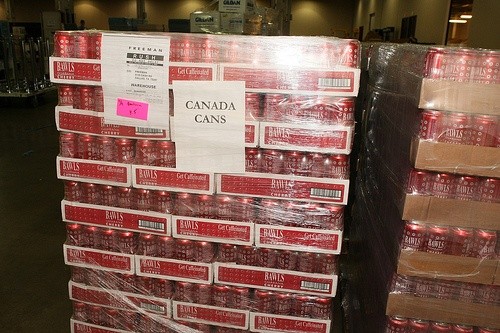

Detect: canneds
[53,30,362,333]
[387,47,500,333]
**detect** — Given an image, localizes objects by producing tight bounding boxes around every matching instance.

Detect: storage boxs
[47,0,500,333]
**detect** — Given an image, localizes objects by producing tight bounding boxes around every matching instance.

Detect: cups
[0,33,53,93]
